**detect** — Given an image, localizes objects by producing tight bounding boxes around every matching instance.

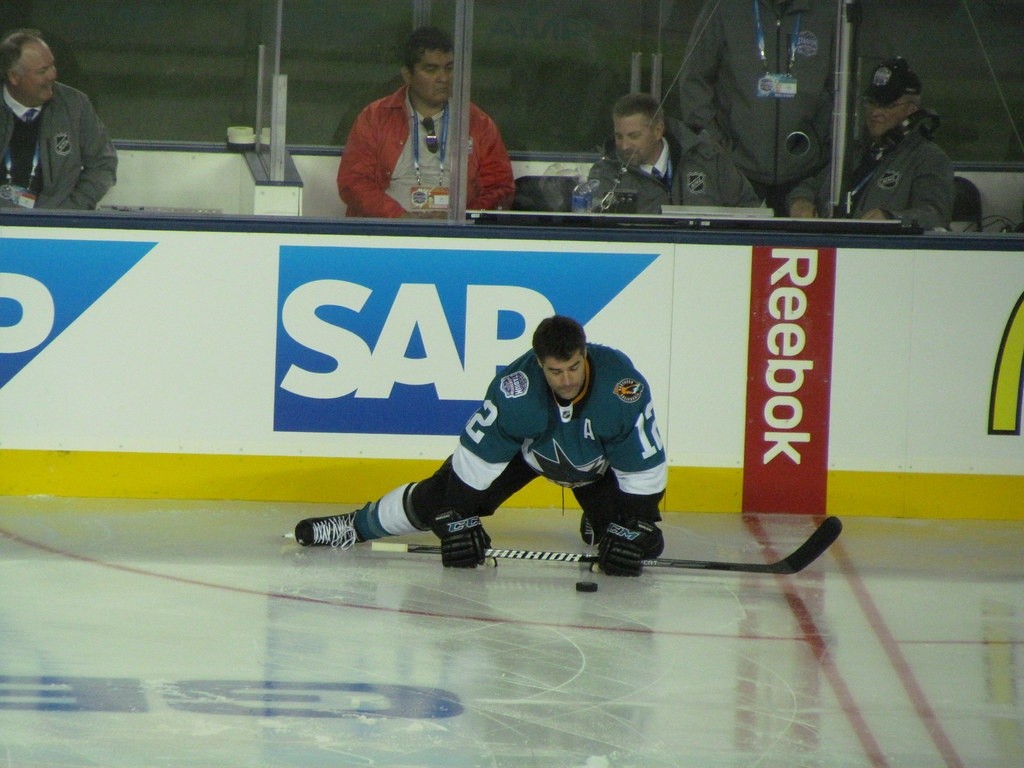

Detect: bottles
[572,176,593,213]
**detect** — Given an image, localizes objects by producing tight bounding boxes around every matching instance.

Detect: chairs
[511,176,580,212]
[952,176,983,232]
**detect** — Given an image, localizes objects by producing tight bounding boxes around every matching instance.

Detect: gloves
[426,502,492,568]
[599,516,661,577]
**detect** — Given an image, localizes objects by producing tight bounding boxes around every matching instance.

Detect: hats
[861,58,921,104]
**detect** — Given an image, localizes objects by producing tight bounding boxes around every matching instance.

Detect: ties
[652,167,663,182]
[24,109,37,123]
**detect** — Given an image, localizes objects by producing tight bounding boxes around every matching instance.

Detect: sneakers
[281,510,368,551]
[580,512,602,545]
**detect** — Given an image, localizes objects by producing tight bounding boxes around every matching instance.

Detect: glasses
[861,101,914,110]
[420,117,438,153]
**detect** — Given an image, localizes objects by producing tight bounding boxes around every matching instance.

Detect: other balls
[575,580,599,593]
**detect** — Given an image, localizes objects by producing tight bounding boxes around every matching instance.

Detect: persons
[576,92,762,215]
[337,24,516,219]
[676,0,848,205]
[0,24,119,211]
[783,54,957,232]
[295,314,668,578]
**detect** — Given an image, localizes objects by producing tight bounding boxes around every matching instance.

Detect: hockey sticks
[371,514,845,575]
